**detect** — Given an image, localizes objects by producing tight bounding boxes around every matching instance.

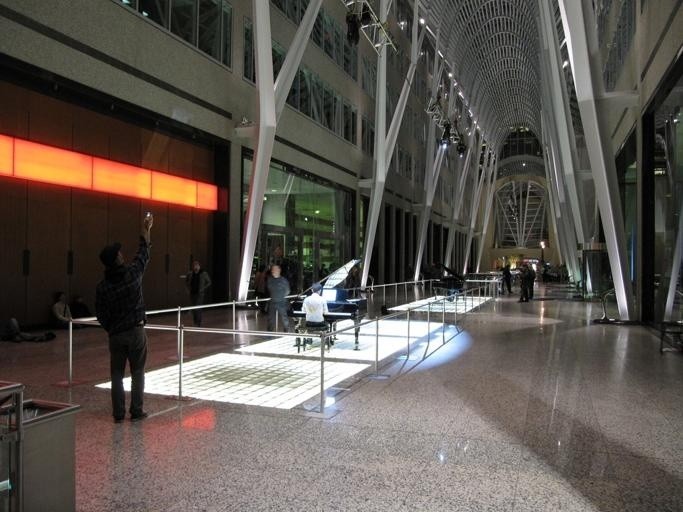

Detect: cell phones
[144,209,151,218]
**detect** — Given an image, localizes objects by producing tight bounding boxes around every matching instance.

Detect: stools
[294,322,331,350]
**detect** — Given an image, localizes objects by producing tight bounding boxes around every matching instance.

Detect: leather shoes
[114,410,147,423]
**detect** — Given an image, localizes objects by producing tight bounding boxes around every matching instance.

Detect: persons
[0,317,57,343]
[301,283,334,348]
[52,292,84,329]
[94,212,154,422]
[68,294,92,318]
[186,257,212,327]
[319,264,329,285]
[348,266,361,297]
[253,245,297,333]
[500,264,549,303]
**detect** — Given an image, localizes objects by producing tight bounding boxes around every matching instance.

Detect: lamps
[343,6,371,48]
[456,130,466,158]
[440,120,452,150]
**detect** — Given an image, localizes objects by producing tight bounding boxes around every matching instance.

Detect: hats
[100,243,121,264]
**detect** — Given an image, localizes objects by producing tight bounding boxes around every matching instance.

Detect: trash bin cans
[0,399,82,512]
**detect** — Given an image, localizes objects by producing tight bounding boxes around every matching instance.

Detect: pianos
[433,264,466,302]
[287,259,368,351]
